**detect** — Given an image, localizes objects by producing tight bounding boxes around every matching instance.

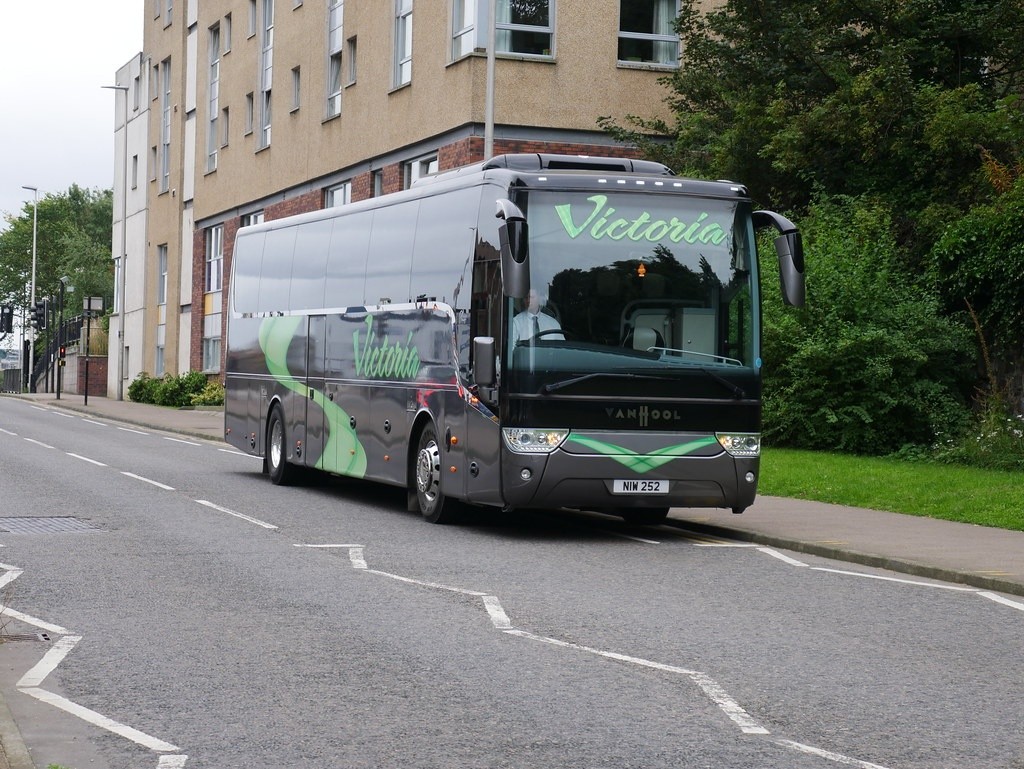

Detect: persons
[513,288,564,350]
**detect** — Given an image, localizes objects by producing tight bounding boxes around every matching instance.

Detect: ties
[532,316,540,340]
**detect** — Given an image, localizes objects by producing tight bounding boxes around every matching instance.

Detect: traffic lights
[59,361,66,366]
[31,306,42,333]
[58,347,66,358]
[35,298,49,330]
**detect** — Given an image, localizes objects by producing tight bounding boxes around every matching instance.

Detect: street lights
[21,185,37,392]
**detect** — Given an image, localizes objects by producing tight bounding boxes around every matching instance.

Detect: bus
[222,153,806,530]
